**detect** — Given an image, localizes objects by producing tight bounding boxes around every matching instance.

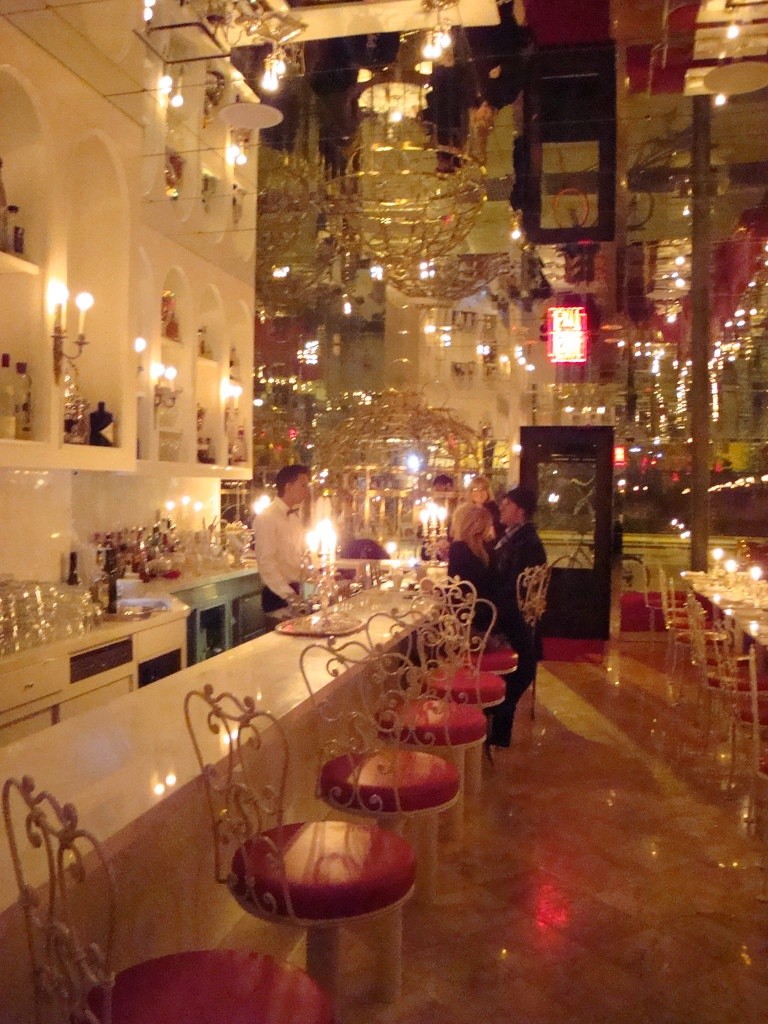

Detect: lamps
[262,0,521,355]
[49,280,93,385]
[154,363,182,409]
[225,386,242,422]
[275,516,366,637]
[414,503,449,568]
[226,131,246,165]
[159,63,184,107]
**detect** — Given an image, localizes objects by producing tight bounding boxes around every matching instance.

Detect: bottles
[0,352,15,438]
[94,520,172,612]
[197,328,207,357]
[205,437,216,462]
[0,159,12,249]
[197,438,206,462]
[16,362,33,439]
[91,400,114,446]
[8,207,26,254]
[232,427,247,462]
[230,347,240,378]
[68,551,77,585]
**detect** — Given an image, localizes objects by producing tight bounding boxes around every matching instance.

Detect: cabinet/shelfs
[0,0,260,747]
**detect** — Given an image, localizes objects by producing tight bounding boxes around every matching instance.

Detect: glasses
[471,487,486,492]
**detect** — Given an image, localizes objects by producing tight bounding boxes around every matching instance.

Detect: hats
[504,488,538,513]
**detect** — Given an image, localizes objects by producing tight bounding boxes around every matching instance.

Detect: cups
[0,579,100,655]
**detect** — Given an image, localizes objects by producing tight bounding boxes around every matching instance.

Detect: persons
[418,475,455,562]
[465,476,500,535]
[485,485,547,581]
[254,464,319,626]
[447,503,538,769]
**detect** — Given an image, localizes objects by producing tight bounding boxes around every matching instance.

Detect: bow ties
[287,508,300,515]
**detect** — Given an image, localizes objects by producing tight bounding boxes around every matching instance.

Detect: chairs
[641,562,768,822]
[409,585,506,848]
[420,573,518,798]
[183,682,416,1024]
[1,775,333,1024]
[366,606,488,905]
[299,636,460,1006]
